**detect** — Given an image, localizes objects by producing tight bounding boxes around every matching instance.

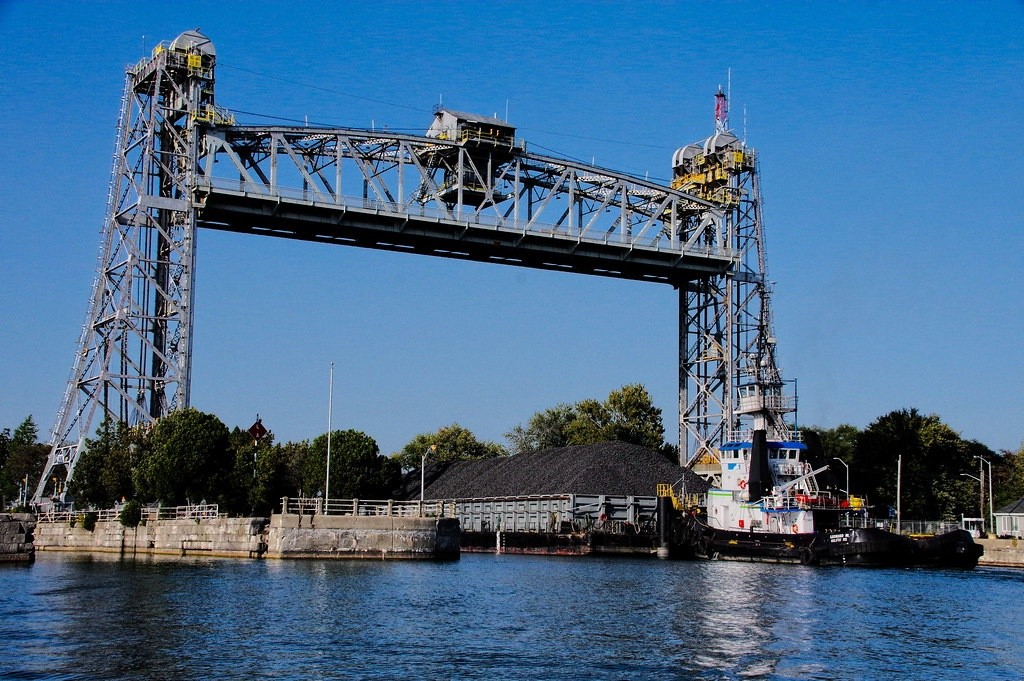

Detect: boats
[694,427,985,570]
[587,517,662,560]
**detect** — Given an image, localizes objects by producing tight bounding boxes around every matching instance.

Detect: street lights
[52,477,57,496]
[973,455,993,534]
[22,473,29,508]
[420,443,438,500]
[959,470,984,532]
[834,457,850,527]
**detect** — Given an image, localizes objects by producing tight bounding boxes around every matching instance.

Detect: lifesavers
[740,480,747,490]
[793,523,799,533]
